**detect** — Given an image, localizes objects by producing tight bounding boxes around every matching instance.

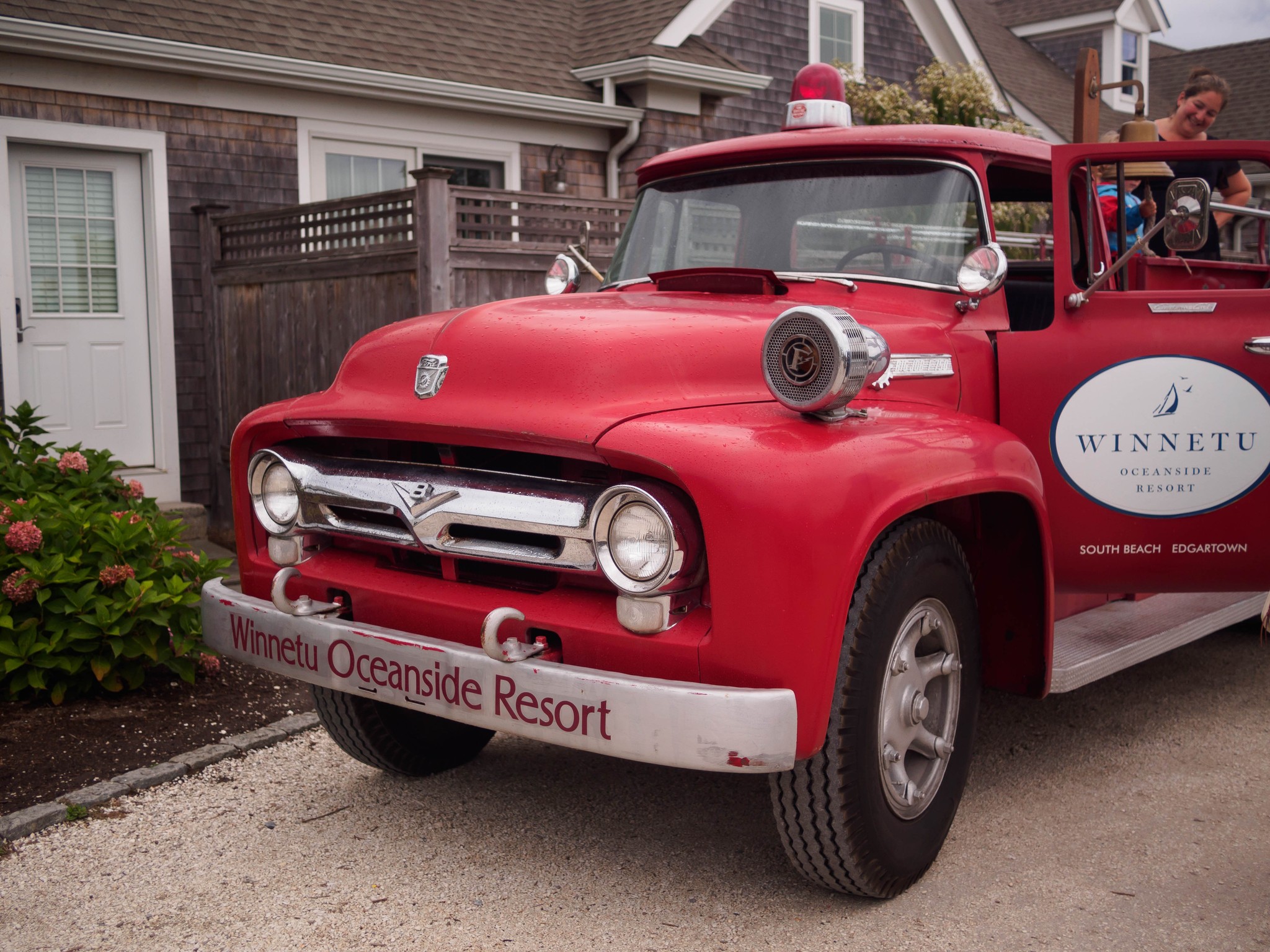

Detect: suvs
[196,60,1269,906]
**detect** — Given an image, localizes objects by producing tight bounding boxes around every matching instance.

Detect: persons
[1131,69,1251,260]
[1092,131,1157,255]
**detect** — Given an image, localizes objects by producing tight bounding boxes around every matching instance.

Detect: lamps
[542,143,571,195]
